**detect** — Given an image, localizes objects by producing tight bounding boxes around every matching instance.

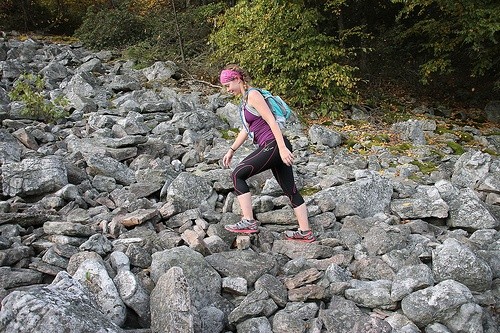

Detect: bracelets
[230,147,236,152]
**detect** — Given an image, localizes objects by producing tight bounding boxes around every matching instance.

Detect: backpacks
[244,88,301,140]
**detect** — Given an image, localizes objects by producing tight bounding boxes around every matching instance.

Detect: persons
[220,64,317,242]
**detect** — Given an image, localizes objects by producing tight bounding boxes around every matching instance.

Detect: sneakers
[224,217,259,232]
[285,229,315,242]
